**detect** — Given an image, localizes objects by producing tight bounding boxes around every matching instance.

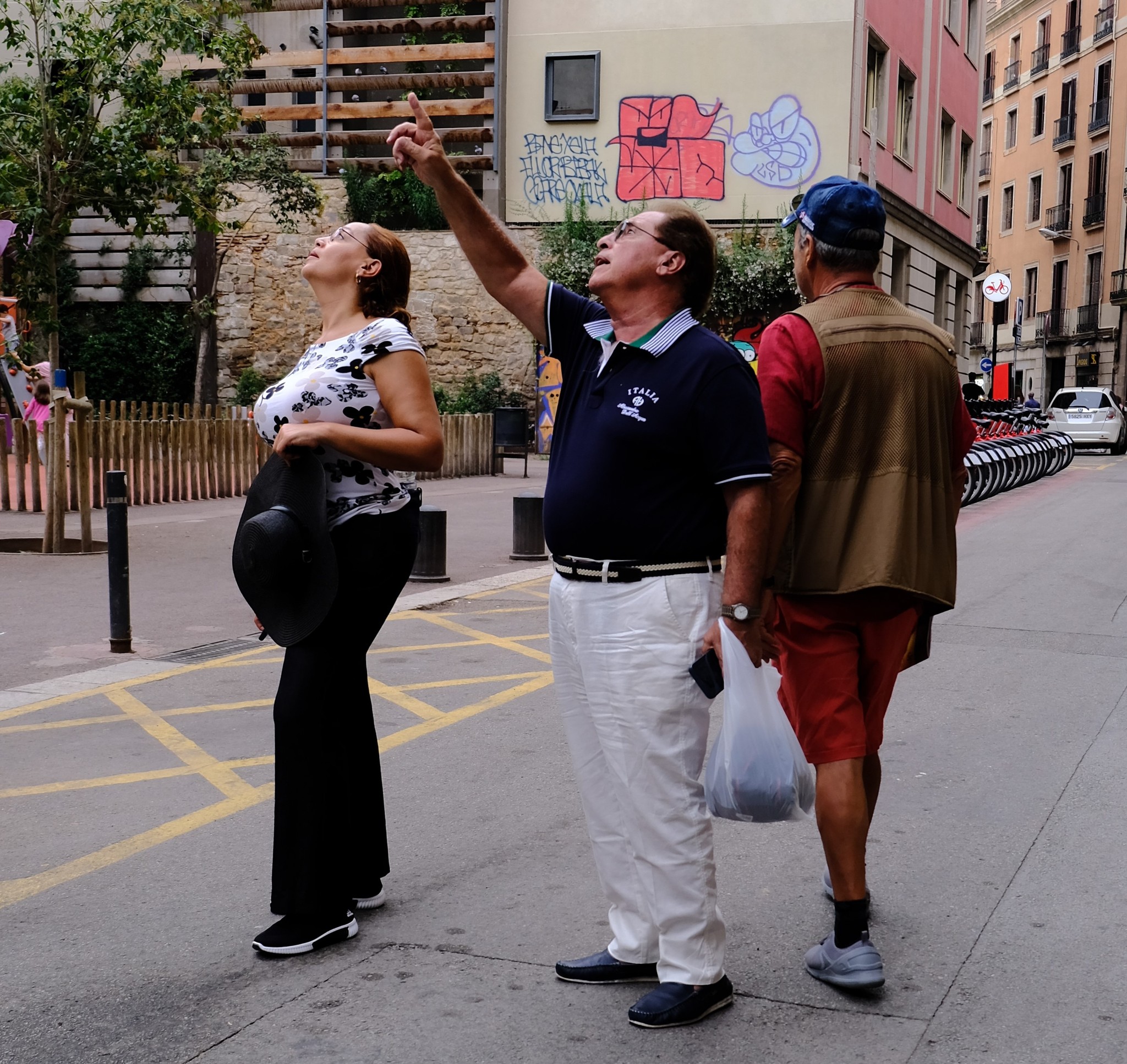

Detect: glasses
[789,194,806,229]
[331,226,371,251]
[614,218,668,246]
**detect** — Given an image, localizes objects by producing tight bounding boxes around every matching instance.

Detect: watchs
[720,603,759,623]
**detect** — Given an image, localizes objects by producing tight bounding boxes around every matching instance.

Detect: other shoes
[805,930,886,990]
[823,863,871,907]
[350,878,385,909]
[555,947,661,983]
[252,914,359,956]
[628,974,733,1028]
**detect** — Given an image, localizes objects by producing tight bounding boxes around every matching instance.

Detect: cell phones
[688,647,724,699]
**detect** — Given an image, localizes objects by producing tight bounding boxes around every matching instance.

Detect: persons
[751,176,977,987]
[961,372,1040,410]
[242,221,443,955]
[0,302,73,469]
[386,90,772,1031]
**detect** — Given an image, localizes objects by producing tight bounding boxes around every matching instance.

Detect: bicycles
[960,397,1075,509]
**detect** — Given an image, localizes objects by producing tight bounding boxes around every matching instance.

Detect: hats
[781,175,887,250]
[232,444,328,646]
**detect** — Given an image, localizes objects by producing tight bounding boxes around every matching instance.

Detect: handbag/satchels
[705,617,818,826]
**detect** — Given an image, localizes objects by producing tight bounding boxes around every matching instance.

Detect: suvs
[1047,386,1127,456]
